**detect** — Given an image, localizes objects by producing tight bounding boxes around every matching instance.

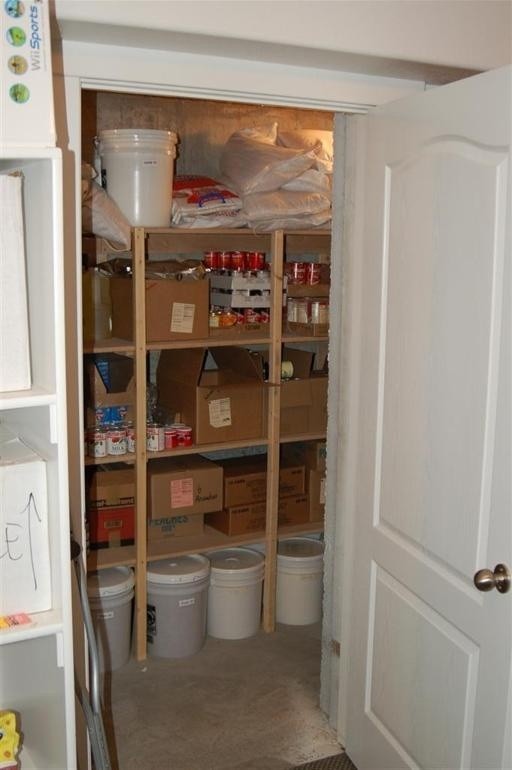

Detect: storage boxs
[2,0,59,149]
[312,376,327,431]
[146,514,205,543]
[307,469,325,524]
[205,494,310,537]
[262,345,314,438]
[155,346,263,444]
[85,462,137,548]
[108,276,209,342]
[2,431,51,620]
[280,443,326,472]
[86,406,137,426]
[148,455,223,520]
[214,457,305,509]
[87,353,138,408]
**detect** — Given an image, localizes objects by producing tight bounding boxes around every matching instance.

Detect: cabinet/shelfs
[274,228,329,630]
[0,149,73,768]
[141,231,278,656]
[79,230,142,664]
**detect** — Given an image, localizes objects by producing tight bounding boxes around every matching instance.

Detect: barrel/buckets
[202,545,265,641]
[87,565,137,672]
[93,128,179,228]
[274,536,325,627]
[146,553,212,659]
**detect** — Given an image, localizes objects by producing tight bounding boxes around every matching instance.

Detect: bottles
[223,312,237,327]
[265,361,293,378]
[84,429,88,456]
[284,262,292,281]
[297,297,312,323]
[88,428,107,457]
[213,270,270,296]
[127,426,136,453]
[217,311,223,326]
[305,262,320,285]
[171,423,186,430]
[106,427,127,454]
[291,262,306,285]
[176,426,192,446]
[311,297,327,324]
[287,298,297,322]
[164,428,176,449]
[147,425,165,452]
[231,250,247,272]
[249,251,266,271]
[209,312,220,327]
[218,250,231,269]
[203,249,218,268]
[235,308,269,324]
